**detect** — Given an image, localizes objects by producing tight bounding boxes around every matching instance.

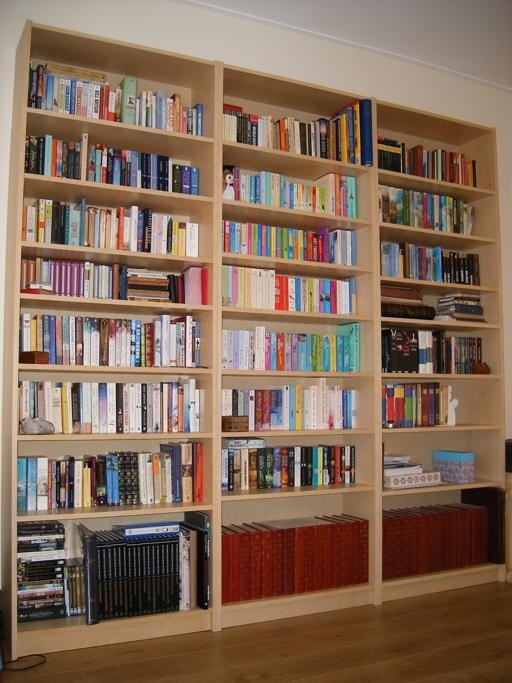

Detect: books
[19,314,201,367]
[377,184,476,235]
[66,512,212,623]
[382,381,458,429]
[18,519,65,622]
[383,441,443,489]
[233,168,356,218]
[221,265,356,314]
[19,377,205,434]
[377,134,478,188]
[382,487,507,583]
[25,132,199,196]
[381,328,491,373]
[17,441,203,512]
[29,62,204,137]
[381,240,481,286]
[381,283,436,320]
[221,377,357,433]
[20,258,209,306]
[223,99,373,168]
[223,219,357,268]
[22,197,200,258]
[435,288,487,323]
[221,438,357,492]
[221,513,369,604]
[221,323,360,372]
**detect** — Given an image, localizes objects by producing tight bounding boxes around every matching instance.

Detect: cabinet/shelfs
[377,99,511,596]
[2,14,217,661]
[218,63,376,628]
